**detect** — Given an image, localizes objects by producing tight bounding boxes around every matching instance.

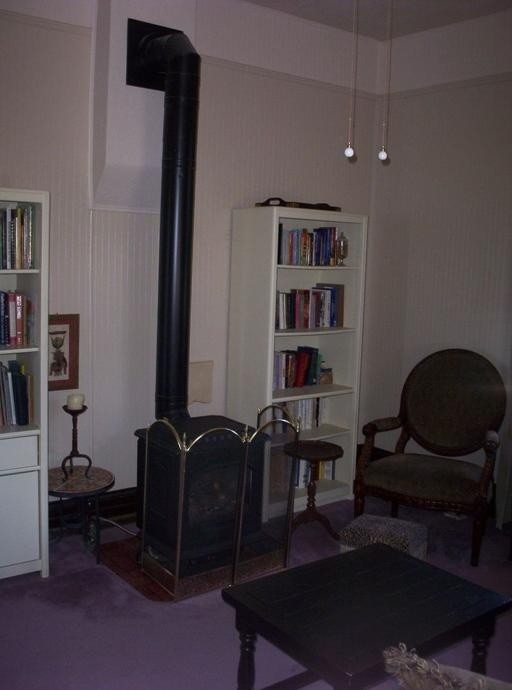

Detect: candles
[67,394,85,410]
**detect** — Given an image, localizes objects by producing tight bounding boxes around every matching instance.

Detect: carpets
[97,534,288,601]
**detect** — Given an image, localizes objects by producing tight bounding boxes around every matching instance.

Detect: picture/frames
[48,312,81,390]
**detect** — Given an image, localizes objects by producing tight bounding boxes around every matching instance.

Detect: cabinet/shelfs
[229,205,371,521]
[0,187,50,584]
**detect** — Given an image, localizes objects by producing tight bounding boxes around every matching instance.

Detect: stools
[340,514,428,560]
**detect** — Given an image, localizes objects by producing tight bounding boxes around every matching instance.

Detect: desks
[48,465,115,563]
[284,439,345,540]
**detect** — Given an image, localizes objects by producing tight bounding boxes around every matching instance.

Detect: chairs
[354,349,508,566]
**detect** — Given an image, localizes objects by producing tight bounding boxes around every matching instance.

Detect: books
[272,223,345,497]
[0,202,37,427]
